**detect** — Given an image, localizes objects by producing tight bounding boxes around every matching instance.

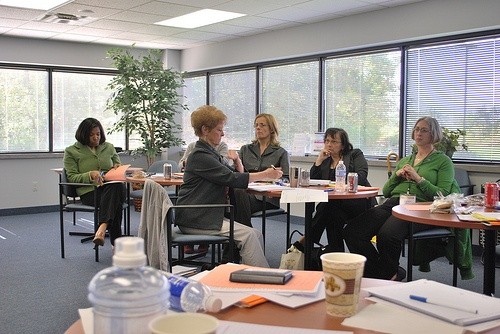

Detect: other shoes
[183,244,210,254]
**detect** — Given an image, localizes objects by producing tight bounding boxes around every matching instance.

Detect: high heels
[93,236,104,250]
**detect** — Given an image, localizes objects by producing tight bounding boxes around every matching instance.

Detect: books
[186,261,325,308]
[369,279,500,326]
[457,212,500,223]
[97,164,144,187]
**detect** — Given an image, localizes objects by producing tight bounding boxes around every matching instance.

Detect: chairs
[52,168,477,286]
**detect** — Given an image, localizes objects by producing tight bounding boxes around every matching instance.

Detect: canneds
[484,182,499,208]
[347,173,358,193]
[300,170,310,187]
[163,163,172,179]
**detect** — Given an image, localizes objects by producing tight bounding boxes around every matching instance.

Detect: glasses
[412,127,431,133]
[324,138,342,145]
[253,123,269,128]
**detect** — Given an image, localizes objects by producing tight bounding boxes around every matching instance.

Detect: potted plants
[411,127,469,159]
[103,43,189,212]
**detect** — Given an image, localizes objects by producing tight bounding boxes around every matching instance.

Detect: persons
[293,127,378,253]
[222,113,290,264]
[174,104,270,269]
[343,115,454,281]
[177,137,229,253]
[63,118,127,249]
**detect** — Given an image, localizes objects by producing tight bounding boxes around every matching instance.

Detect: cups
[320,252,367,318]
[148,313,220,334]
[289,166,299,187]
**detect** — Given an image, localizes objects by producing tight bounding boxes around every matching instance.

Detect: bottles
[87,235,171,334]
[335,161,347,192]
[159,270,222,314]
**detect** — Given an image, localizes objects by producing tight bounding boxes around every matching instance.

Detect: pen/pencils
[97,171,104,183]
[409,294,477,314]
[324,189,333,192]
[271,165,276,170]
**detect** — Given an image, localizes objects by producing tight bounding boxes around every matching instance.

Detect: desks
[392,198,500,296]
[241,178,379,265]
[126,172,189,247]
[63,264,500,334]
